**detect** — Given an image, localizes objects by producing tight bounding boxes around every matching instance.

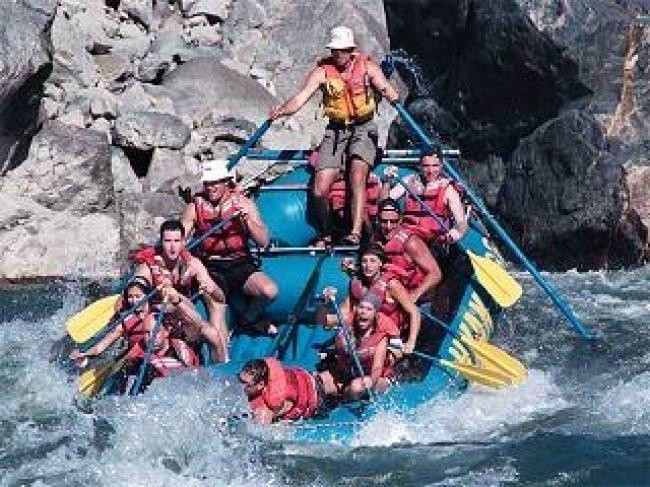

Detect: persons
[379,144,470,246]
[180,160,279,336]
[69,276,222,395]
[342,198,444,304]
[340,242,421,357]
[269,25,400,247]
[239,357,329,425]
[316,286,403,402]
[133,219,229,363]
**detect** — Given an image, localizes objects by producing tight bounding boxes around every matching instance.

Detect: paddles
[417,306,528,378]
[391,343,513,390]
[65,211,242,346]
[72,288,208,397]
[392,173,522,306]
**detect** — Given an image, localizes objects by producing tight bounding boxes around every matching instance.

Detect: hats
[201,161,234,181]
[325,26,358,50]
[378,198,401,212]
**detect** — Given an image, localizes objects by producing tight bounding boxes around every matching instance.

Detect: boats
[88,152,505,442]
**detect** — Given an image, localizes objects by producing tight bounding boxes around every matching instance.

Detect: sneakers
[308,233,360,249]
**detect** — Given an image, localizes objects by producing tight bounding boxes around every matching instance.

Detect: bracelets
[172,297,182,306]
